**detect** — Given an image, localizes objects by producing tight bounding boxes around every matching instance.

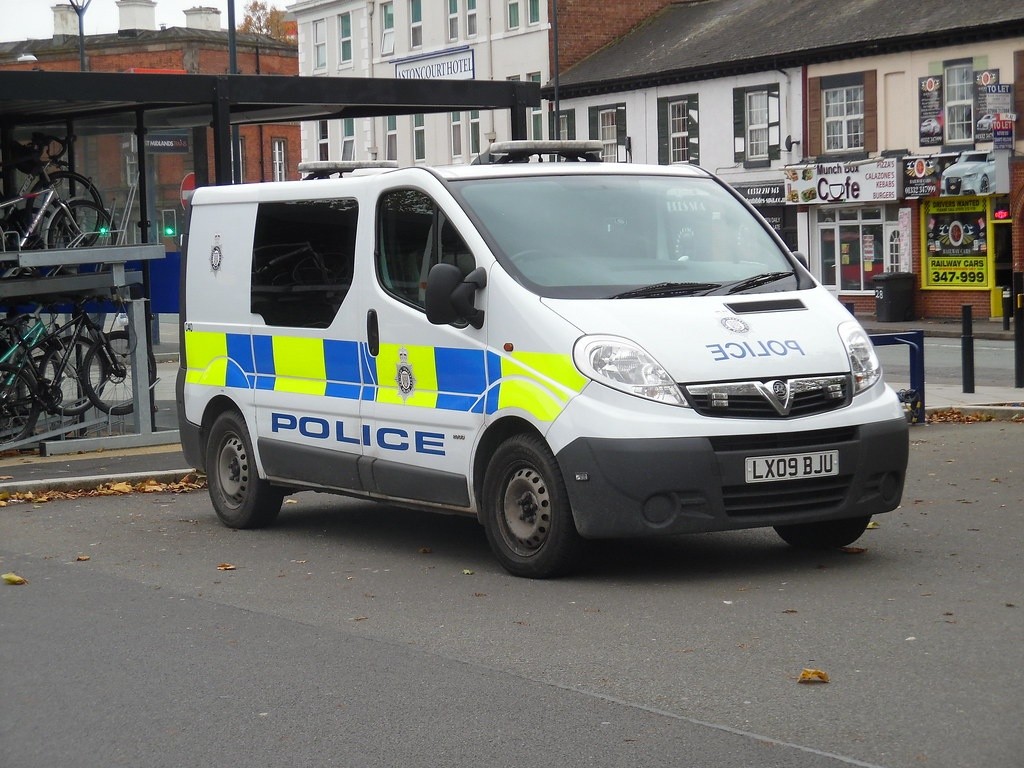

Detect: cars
[939,149,996,198]
[920,118,941,137]
[977,114,996,133]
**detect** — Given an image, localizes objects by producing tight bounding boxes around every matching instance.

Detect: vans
[175,140,908,582]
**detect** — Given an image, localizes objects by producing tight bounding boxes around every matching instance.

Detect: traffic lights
[96,208,112,238]
[161,209,177,238]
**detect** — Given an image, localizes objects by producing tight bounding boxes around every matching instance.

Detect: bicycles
[0,135,156,445]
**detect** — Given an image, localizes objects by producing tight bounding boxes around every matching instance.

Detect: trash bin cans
[872,272,917,323]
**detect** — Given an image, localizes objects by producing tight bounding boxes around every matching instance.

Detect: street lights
[68,0,91,71]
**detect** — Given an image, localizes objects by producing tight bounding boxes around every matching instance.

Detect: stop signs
[179,172,197,211]
